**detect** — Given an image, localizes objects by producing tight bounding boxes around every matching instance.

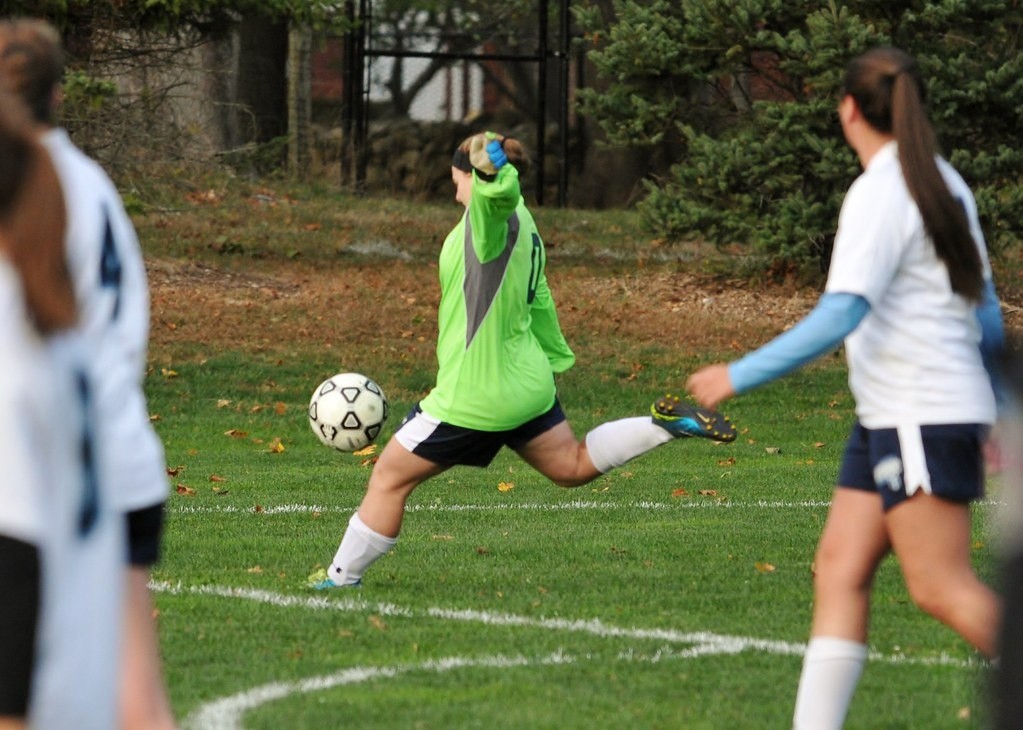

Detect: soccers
[308,372,390,453]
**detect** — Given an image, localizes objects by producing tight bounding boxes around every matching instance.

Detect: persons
[686,45,1001,730]
[299,131,737,591]
[0,121,125,730]
[0,18,177,730]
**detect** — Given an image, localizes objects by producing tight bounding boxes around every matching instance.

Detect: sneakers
[652,391,739,447]
[301,566,361,592]
